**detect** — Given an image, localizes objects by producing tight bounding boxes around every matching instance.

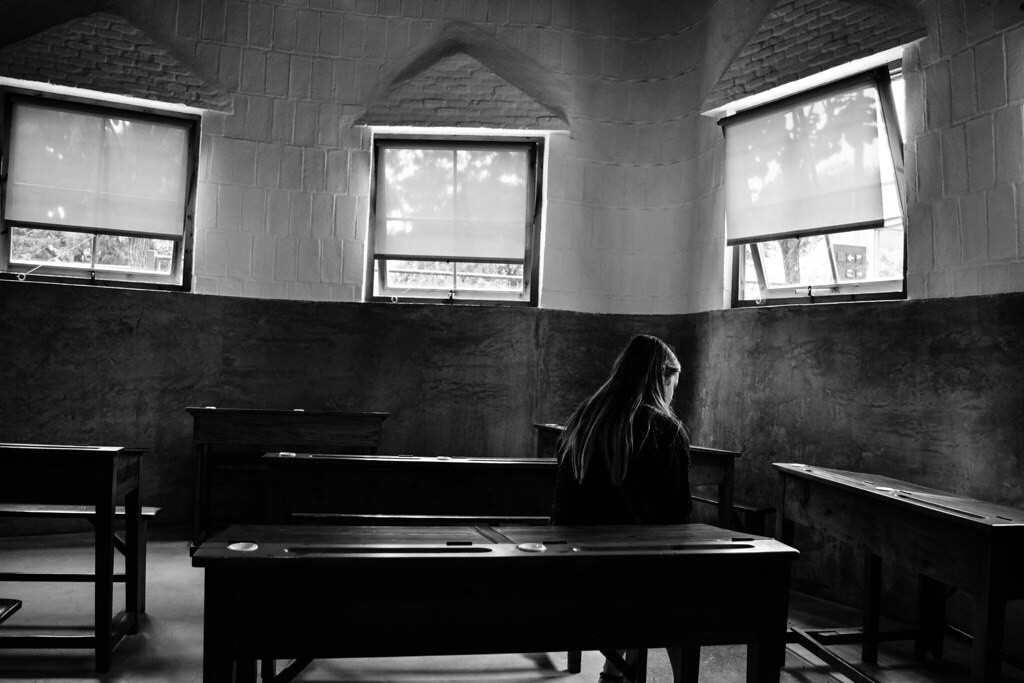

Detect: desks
[770,462,1024,683]
[0,442,151,677]
[191,523,800,683]
[259,451,582,683]
[185,406,392,556]
[534,422,741,530]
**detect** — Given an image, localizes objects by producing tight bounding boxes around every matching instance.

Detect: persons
[547,331,691,683]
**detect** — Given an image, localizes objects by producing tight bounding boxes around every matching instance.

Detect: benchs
[1,502,162,616]
[691,496,776,537]
[218,464,272,525]
